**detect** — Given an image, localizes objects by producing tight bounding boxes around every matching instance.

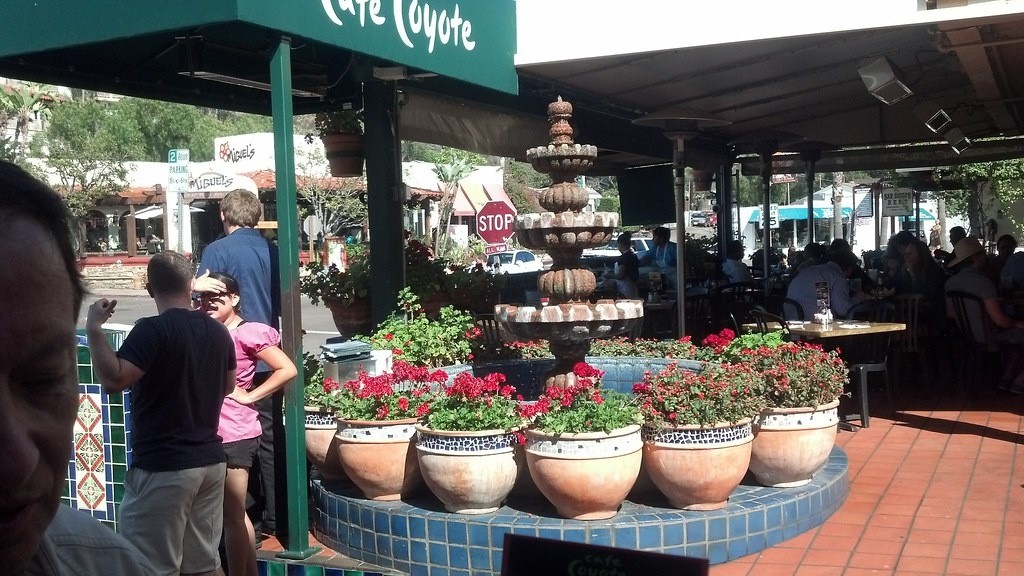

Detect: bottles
[878,276,883,297]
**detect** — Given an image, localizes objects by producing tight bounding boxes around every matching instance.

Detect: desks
[741,321,907,420]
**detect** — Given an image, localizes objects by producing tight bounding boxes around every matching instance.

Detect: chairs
[477,266,1012,427]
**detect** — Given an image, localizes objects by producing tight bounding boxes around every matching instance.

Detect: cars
[582,237,654,257]
[467,250,544,276]
[690,210,718,227]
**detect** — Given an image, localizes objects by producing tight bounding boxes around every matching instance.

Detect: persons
[86,250,238,576]
[602,223,1024,395]
[191,189,286,550]
[190,269,297,576]
[0,159,163,576]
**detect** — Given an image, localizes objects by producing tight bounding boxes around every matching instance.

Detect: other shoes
[1009,383,1024,395]
[997,383,1009,391]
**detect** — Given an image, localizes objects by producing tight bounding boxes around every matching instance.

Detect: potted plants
[298,229,499,338]
[305,106,364,177]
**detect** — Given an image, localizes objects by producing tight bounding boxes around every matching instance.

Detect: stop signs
[476,200,516,245]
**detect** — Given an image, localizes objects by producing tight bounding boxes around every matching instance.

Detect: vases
[304,400,839,521]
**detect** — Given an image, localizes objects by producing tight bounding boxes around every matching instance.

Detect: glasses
[196,291,220,302]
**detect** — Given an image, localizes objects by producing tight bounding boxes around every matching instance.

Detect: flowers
[297,285,854,446]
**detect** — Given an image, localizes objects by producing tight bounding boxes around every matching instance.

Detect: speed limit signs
[758,203,779,229]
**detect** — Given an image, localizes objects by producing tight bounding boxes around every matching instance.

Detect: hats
[947,236,986,268]
